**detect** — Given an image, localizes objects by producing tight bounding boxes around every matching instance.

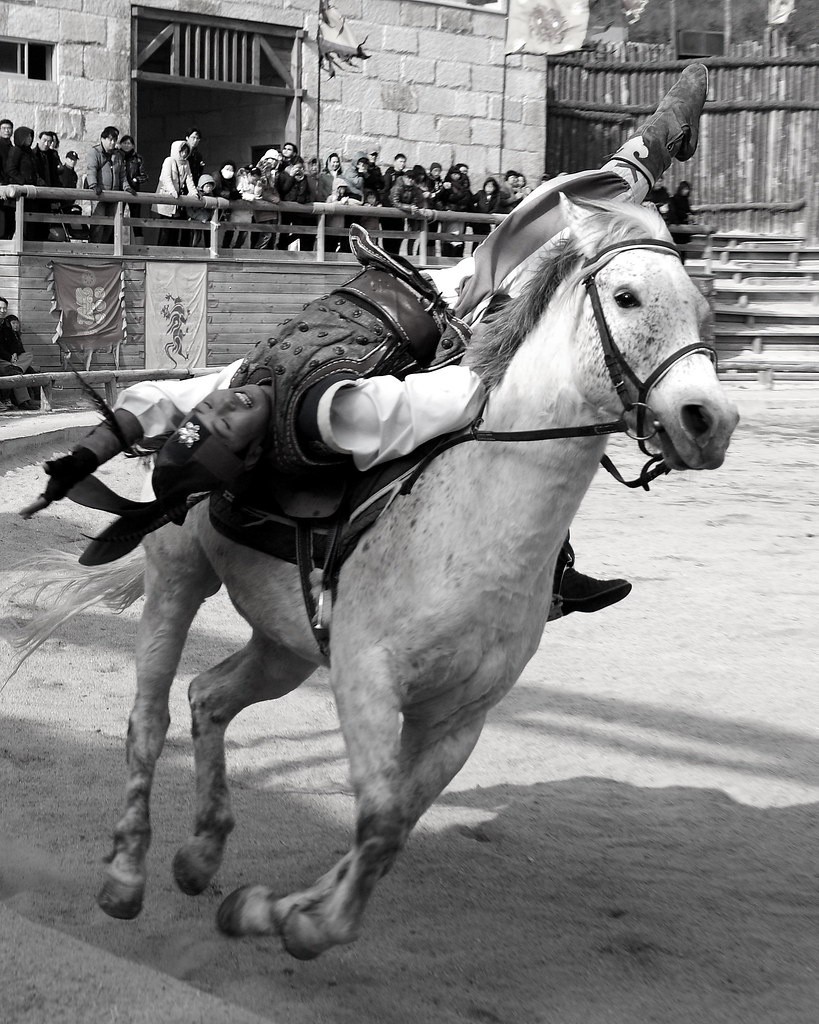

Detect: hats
[366,148,378,156]
[264,149,282,161]
[430,162,442,172]
[66,151,79,160]
[151,409,236,526]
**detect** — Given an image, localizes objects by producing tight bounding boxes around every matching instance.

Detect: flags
[317,0,372,82]
[44,259,128,372]
[145,261,208,370]
[505,0,589,57]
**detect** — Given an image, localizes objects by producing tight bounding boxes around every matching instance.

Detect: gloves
[93,186,101,195]
[126,188,136,196]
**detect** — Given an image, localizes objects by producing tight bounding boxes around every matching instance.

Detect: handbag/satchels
[84,177,89,189]
[179,186,189,197]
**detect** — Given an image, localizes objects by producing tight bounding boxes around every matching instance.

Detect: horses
[1,187,740,964]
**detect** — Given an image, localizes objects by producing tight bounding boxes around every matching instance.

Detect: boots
[546,568,631,621]
[608,64,709,194]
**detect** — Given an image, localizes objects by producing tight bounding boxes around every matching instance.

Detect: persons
[641,175,700,265]
[18,62,711,620]
[0,119,552,258]
[0,297,40,410]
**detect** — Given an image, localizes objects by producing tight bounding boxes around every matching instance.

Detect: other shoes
[19,399,42,411]
